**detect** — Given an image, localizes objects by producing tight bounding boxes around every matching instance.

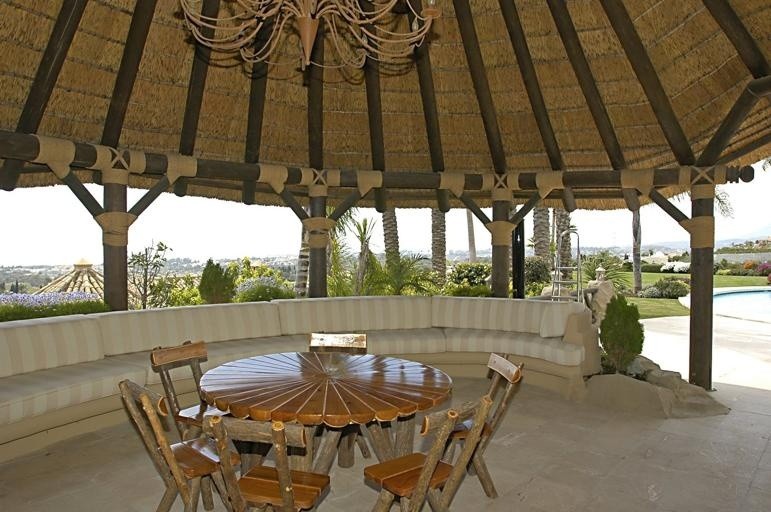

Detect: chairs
[154,341,210,440]
[310,334,366,355]
[120,379,242,512]
[445,351,524,498]
[363,396,493,510]
[204,415,331,512]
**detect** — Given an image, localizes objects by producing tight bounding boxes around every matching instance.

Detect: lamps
[178,0,444,77]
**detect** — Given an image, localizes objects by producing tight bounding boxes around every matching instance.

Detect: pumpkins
[552,229,584,303]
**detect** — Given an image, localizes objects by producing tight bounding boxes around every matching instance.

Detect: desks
[198,353,453,474]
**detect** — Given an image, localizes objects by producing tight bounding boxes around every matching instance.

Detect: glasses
[178,0,444,77]
